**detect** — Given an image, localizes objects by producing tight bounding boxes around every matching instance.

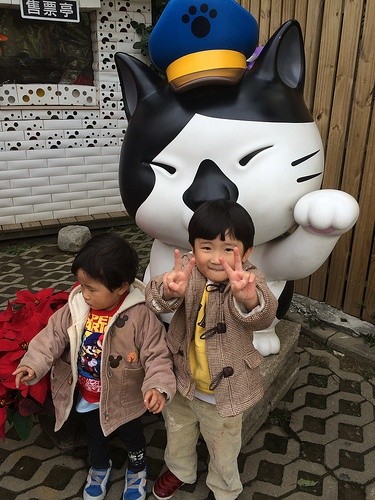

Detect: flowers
[0,287,68,444]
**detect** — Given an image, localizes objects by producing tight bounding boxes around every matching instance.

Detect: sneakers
[83,460,112,500]
[122,464,148,500]
[152,468,187,500]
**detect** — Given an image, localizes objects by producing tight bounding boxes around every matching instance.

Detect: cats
[114,19,361,358]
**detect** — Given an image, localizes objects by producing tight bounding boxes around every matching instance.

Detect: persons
[11,233,177,500]
[144,199,278,500]
